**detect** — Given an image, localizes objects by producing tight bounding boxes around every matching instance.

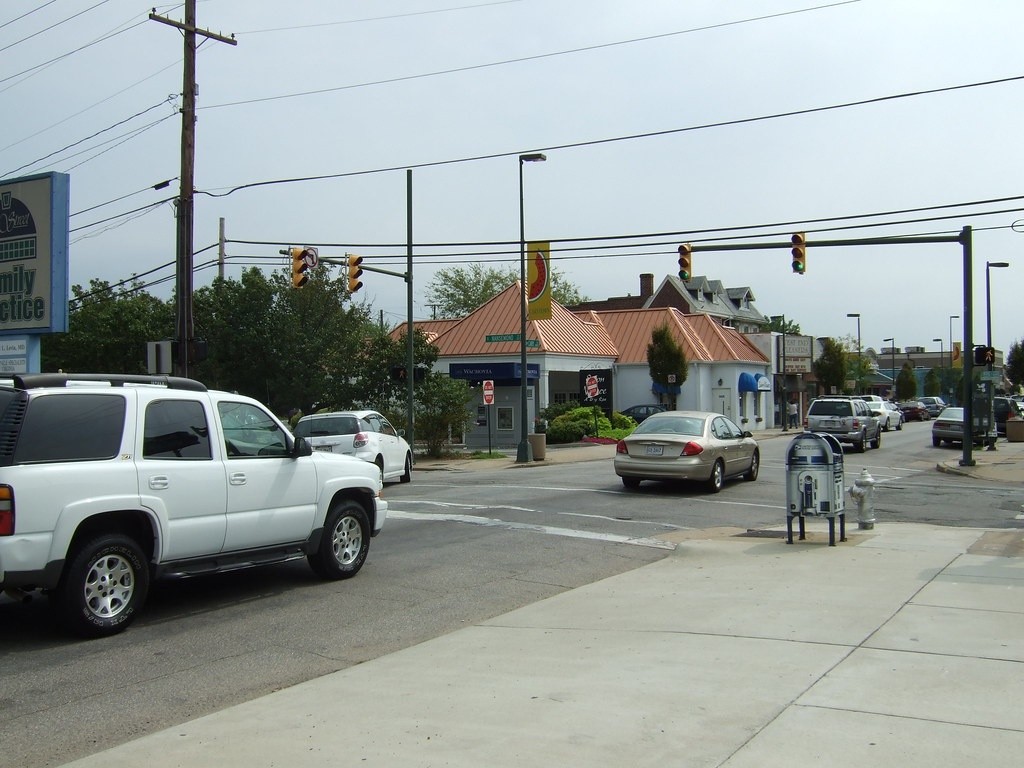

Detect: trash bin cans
[786,429,846,547]
[534,425,546,433]
[527,433,546,461]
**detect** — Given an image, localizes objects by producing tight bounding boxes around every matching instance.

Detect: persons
[788,400,798,429]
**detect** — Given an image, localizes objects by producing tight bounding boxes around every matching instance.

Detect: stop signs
[483,380,494,405]
[585,375,599,398]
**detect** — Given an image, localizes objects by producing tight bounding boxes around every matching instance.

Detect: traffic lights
[292,248,308,288]
[791,233,805,273]
[974,348,995,364]
[677,244,691,282]
[348,253,363,293]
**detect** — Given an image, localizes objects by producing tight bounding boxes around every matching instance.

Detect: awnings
[755,374,772,392]
[739,373,758,392]
[774,374,808,392]
[652,383,681,394]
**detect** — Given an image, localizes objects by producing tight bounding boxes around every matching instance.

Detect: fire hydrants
[849,467,877,530]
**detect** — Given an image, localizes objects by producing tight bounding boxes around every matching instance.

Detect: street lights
[950,316,959,388]
[516,153,548,463]
[986,260,1010,450]
[846,313,863,395]
[771,314,789,431]
[883,337,895,404]
[932,338,943,392]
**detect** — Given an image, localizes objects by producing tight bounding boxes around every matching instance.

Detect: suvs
[0,372,388,641]
[802,394,882,453]
[993,396,1019,429]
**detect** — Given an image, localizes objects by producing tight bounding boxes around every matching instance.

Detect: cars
[932,406,984,446]
[620,404,693,429]
[900,401,931,421]
[613,411,761,493]
[293,411,414,484]
[861,395,905,432]
[1010,394,1024,411]
[919,397,946,417]
